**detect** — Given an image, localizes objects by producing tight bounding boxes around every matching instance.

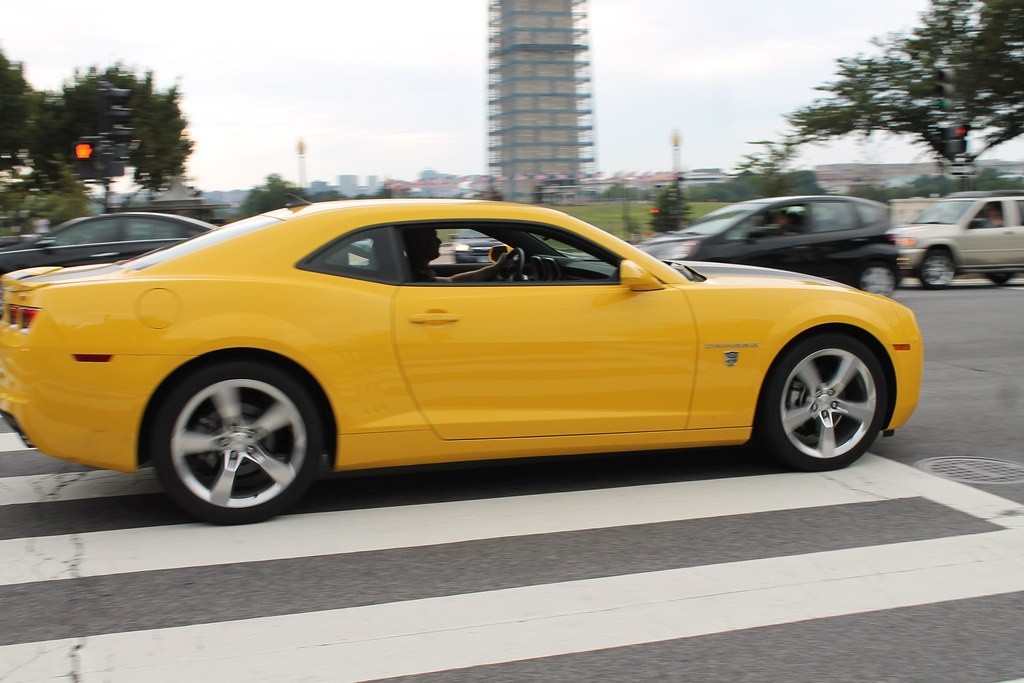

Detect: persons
[401,228,513,282]
[766,212,795,236]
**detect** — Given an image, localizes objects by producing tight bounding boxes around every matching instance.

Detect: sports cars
[0,199,928,526]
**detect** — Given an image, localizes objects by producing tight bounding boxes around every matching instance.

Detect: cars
[641,195,901,301]
[0,211,226,279]
[881,188,1024,291]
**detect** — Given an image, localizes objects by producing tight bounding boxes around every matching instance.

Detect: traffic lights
[72,134,104,172]
[94,78,136,152]
[933,63,965,114]
[937,118,971,157]
